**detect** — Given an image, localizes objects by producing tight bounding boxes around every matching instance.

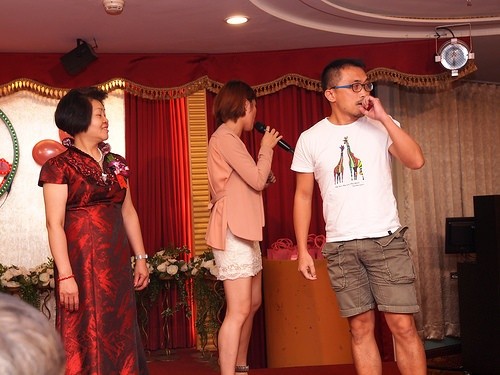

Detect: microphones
[253,122,294,154]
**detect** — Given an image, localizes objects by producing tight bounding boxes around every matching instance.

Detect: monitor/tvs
[444,217,475,254]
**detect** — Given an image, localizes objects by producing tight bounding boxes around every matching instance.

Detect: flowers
[131,246,219,337]
[107,152,135,177]
[0,256,57,310]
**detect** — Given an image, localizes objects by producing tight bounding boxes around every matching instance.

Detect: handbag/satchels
[266,234,326,260]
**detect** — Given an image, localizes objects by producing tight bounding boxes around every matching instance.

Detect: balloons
[58,128,74,142]
[33,139,67,165]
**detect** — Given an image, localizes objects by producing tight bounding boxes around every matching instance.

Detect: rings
[264,130,270,132]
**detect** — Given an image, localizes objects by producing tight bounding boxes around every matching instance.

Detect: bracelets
[57,274,74,281]
[134,254,150,259]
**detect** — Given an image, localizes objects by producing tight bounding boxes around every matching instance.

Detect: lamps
[59,37,97,76]
[434,21,474,77]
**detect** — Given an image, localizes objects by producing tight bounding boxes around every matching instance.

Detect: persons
[41,85,150,375]
[289,58,427,375]
[204,81,283,374]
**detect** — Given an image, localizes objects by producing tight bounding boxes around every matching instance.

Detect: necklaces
[95,148,103,163]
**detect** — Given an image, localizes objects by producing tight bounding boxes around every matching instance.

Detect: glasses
[329,81,374,93]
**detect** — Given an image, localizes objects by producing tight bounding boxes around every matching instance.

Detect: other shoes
[235,366,250,375]
